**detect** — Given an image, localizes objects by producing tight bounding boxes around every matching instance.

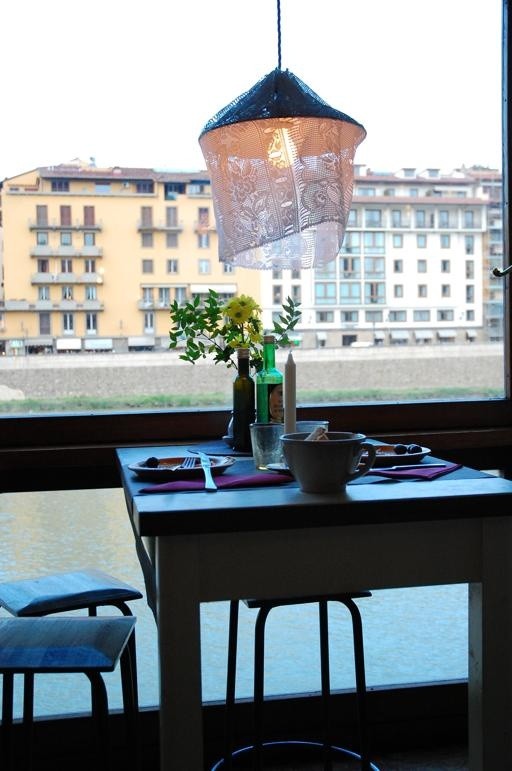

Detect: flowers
[218,294,264,351]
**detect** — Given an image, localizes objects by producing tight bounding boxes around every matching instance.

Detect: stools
[0,616,138,769]
[226,591,372,770]
[1,568,142,770]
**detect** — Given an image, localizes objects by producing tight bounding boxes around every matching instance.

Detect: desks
[116,438,511,769]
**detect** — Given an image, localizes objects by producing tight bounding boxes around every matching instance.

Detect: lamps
[199,2,367,266]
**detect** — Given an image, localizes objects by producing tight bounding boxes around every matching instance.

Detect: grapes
[146,457,159,468]
[394,443,422,454]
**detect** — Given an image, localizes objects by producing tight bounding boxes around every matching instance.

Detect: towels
[364,462,464,481]
[139,473,293,495]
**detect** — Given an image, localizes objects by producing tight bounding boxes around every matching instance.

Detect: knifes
[197,452,217,493]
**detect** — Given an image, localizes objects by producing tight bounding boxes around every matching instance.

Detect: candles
[283,347,297,433]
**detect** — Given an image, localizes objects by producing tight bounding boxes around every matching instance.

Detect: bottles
[256,336,283,424]
[231,348,255,453]
[228,411,235,436]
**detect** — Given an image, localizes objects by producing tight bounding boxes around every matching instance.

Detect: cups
[248,422,284,472]
[295,421,329,433]
[277,431,375,494]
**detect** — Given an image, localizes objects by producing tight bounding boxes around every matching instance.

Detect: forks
[171,457,194,473]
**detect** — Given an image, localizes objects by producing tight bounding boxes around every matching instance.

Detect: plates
[266,461,294,474]
[222,435,233,446]
[126,455,236,481]
[362,444,430,467]
